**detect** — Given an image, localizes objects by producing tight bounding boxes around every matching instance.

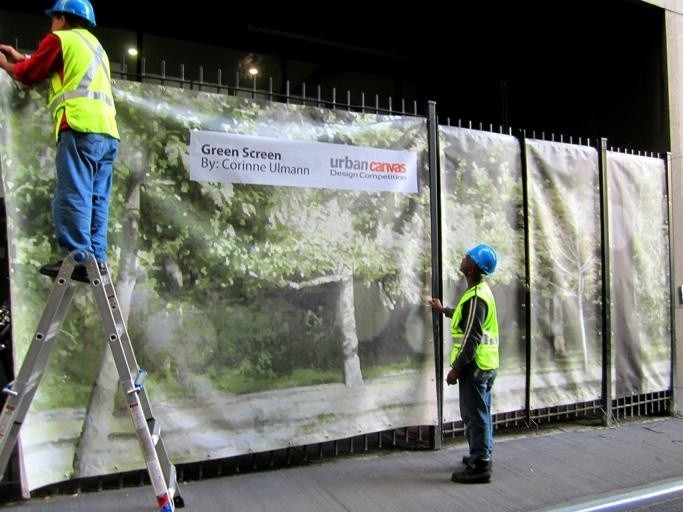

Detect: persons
[0,0,119,282]
[428,242,503,484]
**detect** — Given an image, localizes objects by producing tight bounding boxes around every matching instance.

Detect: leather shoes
[38,259,107,284]
[462,454,492,467]
[450,467,491,484]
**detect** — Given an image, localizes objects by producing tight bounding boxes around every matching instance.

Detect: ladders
[0,249,185,512]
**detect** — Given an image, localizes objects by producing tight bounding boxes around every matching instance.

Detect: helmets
[463,243,497,276]
[44,0,96,30]
